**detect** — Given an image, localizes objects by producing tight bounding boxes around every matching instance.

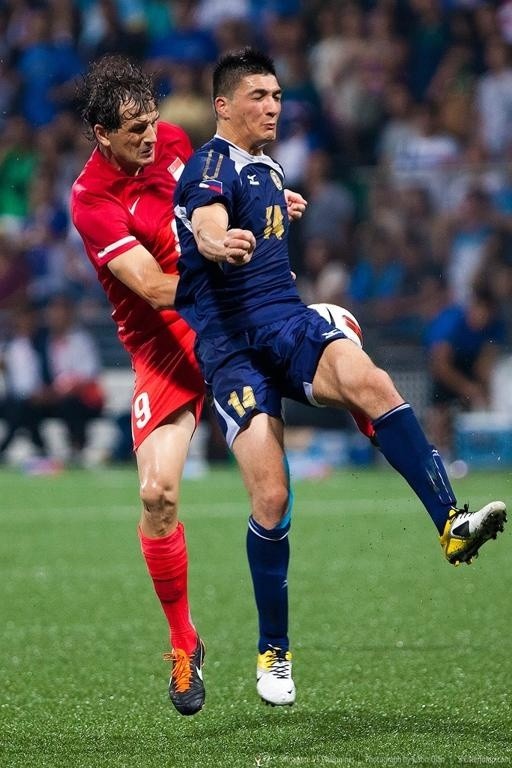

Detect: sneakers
[437,500,508,568]
[256,642,297,707]
[162,631,206,716]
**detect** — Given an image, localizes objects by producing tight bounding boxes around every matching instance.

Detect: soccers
[308,303,363,348]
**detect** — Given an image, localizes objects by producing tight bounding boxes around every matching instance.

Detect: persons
[171,44,506,704]
[1,1,511,478]
[68,53,376,713]
